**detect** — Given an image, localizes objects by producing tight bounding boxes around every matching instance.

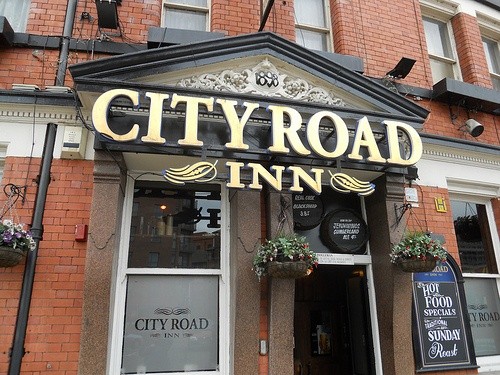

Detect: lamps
[458,118,485,138]
[389,57,417,80]
[93,0,124,38]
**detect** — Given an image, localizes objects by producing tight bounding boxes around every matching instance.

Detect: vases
[401,260,436,274]
[0,219,37,251]
[0,247,24,267]
[266,262,307,280]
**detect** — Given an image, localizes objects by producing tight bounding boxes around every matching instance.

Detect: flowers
[255,234,317,283]
[388,228,447,269]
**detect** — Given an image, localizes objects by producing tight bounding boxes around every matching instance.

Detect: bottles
[165,213,174,236]
[157,215,165,235]
